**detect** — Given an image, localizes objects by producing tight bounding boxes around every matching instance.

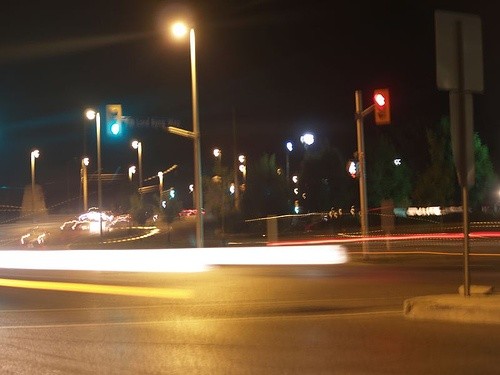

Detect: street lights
[31,150,40,223]
[132,141,142,187]
[239,154,246,184]
[81,158,89,211]
[213,148,221,176]
[86,110,102,237]
[128,166,136,234]
[157,171,162,201]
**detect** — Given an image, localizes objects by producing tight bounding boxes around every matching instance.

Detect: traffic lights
[172,22,203,247]
[374,89,390,125]
[107,104,122,135]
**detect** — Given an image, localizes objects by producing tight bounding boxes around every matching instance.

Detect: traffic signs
[449,91,475,187]
[434,10,483,92]
